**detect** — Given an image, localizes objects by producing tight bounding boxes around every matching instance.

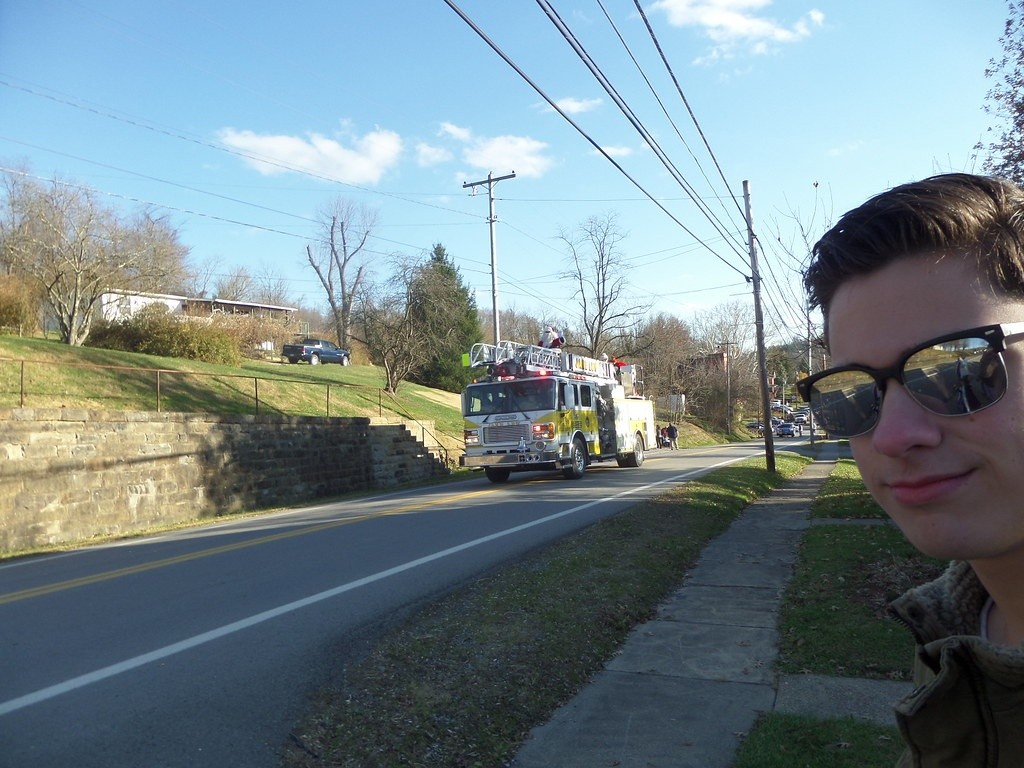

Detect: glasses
[795,322,1024,438]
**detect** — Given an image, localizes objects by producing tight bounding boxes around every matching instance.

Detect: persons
[798,420,817,436]
[655,422,679,450]
[793,172,1024,768]
[538,327,565,348]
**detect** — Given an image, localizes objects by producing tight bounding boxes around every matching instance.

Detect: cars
[745,405,820,437]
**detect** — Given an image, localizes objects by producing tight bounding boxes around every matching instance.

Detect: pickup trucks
[281,338,350,367]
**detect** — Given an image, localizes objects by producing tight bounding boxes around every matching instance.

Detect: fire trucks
[455,338,659,482]
